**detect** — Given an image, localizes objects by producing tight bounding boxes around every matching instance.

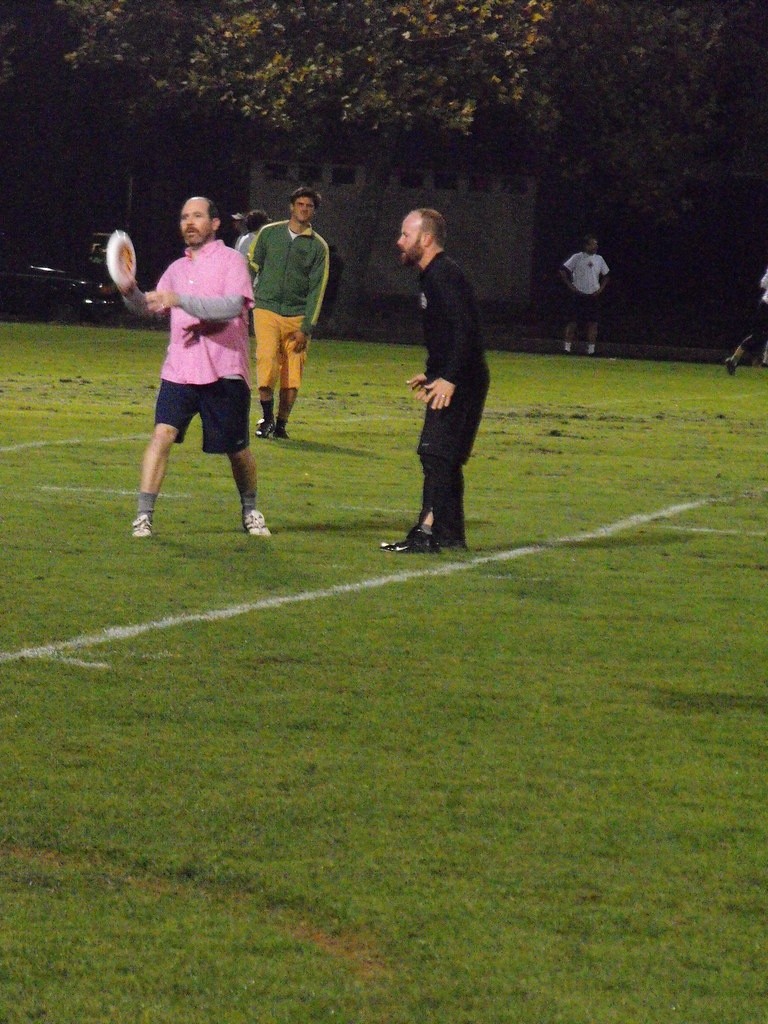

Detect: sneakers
[242,510,272,536]
[273,426,289,439]
[255,419,274,438]
[132,514,152,538]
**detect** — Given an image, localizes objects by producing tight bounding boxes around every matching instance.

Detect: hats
[232,213,245,219]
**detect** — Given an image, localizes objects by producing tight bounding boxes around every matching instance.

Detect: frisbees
[102,226,140,293]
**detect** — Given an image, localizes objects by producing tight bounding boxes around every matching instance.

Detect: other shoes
[564,350,572,355]
[587,351,596,357]
[724,358,738,375]
[759,362,768,370]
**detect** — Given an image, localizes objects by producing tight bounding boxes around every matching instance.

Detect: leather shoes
[379,527,439,553]
[434,535,466,551]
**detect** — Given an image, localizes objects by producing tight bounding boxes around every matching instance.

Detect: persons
[118,196,270,537]
[231,209,344,327]
[247,187,330,440]
[724,269,768,375]
[380,208,492,552]
[559,237,612,357]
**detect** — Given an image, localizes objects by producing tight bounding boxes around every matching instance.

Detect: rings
[161,304,165,309]
[441,394,446,398]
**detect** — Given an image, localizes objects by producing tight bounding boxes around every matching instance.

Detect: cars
[1,227,125,325]
